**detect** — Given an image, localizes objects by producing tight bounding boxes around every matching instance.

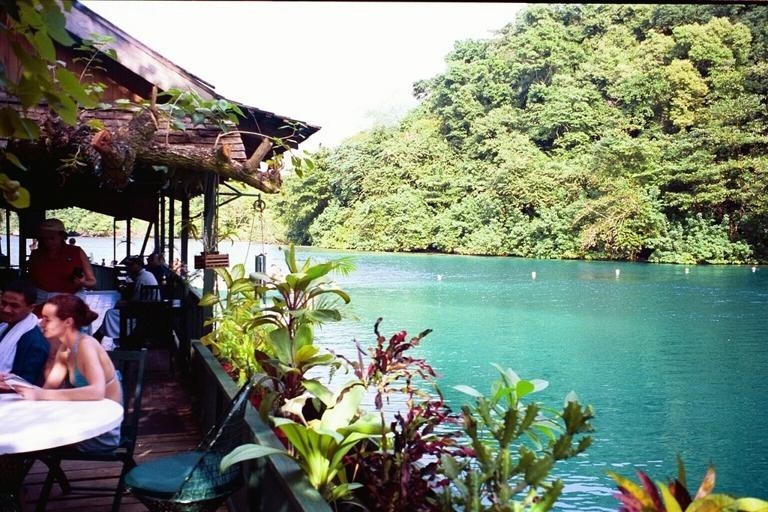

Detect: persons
[2,282,51,392]
[98,254,160,338]
[1,285,125,456]
[147,252,174,284]
[27,218,97,303]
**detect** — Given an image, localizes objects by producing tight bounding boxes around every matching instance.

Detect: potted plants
[182,173,251,269]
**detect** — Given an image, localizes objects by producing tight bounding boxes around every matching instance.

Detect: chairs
[34,350,255,510]
[115,283,177,351]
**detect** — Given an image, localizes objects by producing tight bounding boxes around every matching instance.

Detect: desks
[0,392,124,510]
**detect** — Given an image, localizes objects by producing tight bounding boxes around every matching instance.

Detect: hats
[39,218,65,232]
[125,258,144,268]
